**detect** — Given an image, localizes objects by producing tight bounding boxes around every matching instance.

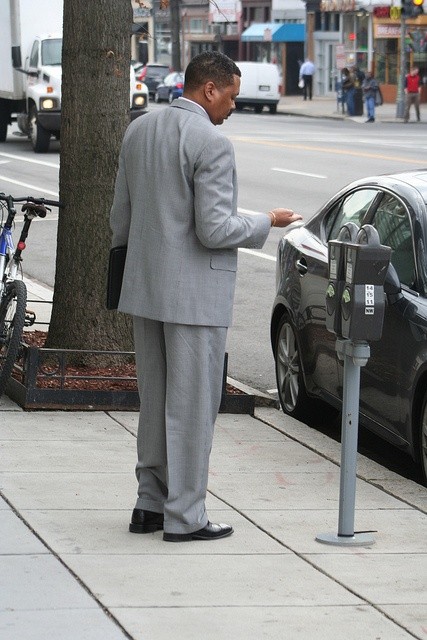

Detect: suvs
[134,64,173,100]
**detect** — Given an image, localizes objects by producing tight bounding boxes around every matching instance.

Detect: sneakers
[366,117,374,123]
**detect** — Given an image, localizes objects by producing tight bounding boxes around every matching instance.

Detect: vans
[234,61,281,113]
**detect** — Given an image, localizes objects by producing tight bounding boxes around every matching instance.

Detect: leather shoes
[127,506,161,533]
[162,521,233,543]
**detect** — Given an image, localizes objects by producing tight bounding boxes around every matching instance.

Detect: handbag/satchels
[373,86,382,106]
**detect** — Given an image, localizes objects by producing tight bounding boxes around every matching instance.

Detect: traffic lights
[403,0,425,20]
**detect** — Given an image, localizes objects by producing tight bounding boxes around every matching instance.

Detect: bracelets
[270,212,276,228]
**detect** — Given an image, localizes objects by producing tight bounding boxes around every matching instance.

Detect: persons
[298,57,314,101]
[361,70,377,123]
[111,51,303,542]
[403,66,419,122]
[341,65,365,117]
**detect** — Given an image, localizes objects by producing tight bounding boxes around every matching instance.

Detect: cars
[154,72,184,102]
[269,167,427,479]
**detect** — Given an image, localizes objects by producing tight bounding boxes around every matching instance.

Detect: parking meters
[325,222,391,537]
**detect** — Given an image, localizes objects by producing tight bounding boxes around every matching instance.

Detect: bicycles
[0,190,59,394]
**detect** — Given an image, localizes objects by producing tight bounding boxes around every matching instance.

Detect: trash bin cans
[346,88,362,116]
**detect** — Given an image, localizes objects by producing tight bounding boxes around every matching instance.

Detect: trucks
[0,1,149,152]
